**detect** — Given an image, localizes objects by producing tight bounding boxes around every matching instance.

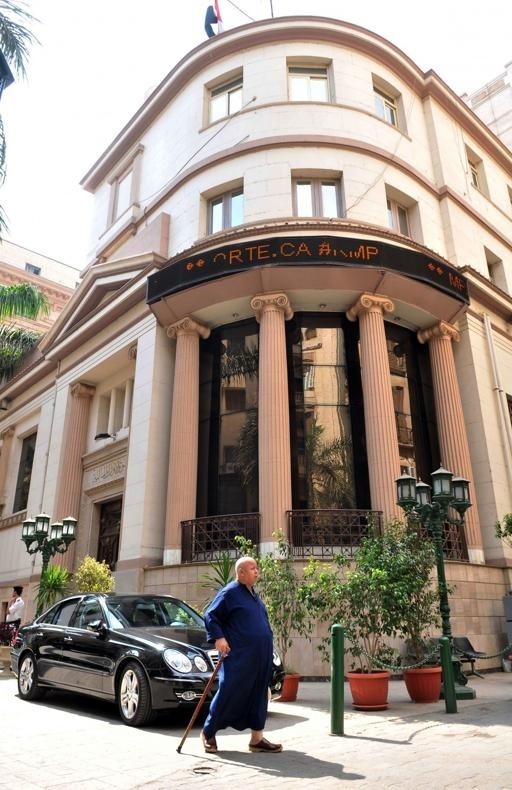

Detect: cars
[10,591,287,726]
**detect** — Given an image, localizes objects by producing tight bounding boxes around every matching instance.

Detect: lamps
[0,397,11,410]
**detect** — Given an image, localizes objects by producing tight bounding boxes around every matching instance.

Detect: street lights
[21,512,77,616]
[393,462,473,713]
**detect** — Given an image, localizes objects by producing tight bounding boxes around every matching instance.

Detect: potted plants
[235,528,299,702]
[300,513,455,710]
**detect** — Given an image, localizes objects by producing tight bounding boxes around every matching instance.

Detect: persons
[5,586,26,647]
[199,556,284,754]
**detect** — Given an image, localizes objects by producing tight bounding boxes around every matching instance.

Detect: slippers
[200,729,217,753]
[248,737,282,753]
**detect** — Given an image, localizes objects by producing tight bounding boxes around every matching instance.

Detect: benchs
[403,636,487,680]
